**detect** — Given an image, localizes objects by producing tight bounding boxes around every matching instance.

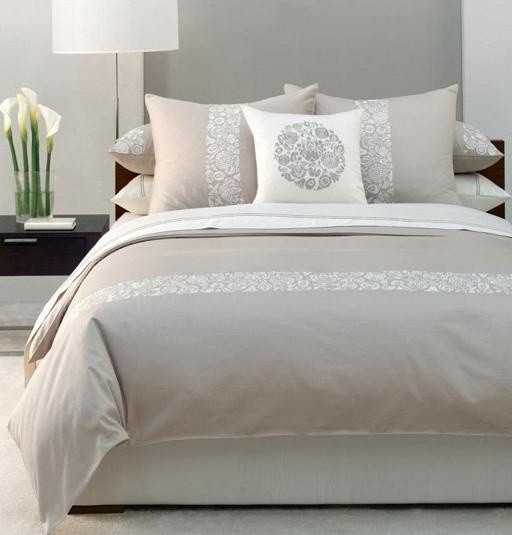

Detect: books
[24,217,77,231]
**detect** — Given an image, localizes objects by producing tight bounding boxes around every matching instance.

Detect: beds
[11,136,512,535]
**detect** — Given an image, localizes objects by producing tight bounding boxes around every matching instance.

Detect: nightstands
[0,214,110,330]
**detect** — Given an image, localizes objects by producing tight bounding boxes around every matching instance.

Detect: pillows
[109,173,154,214]
[107,124,155,176]
[283,82,462,208]
[456,173,511,212]
[239,102,369,204]
[454,116,504,173]
[144,83,320,212]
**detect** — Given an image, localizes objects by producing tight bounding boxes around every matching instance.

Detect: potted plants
[0,87,63,222]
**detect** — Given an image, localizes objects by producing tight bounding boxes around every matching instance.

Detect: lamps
[51,2,178,142]
